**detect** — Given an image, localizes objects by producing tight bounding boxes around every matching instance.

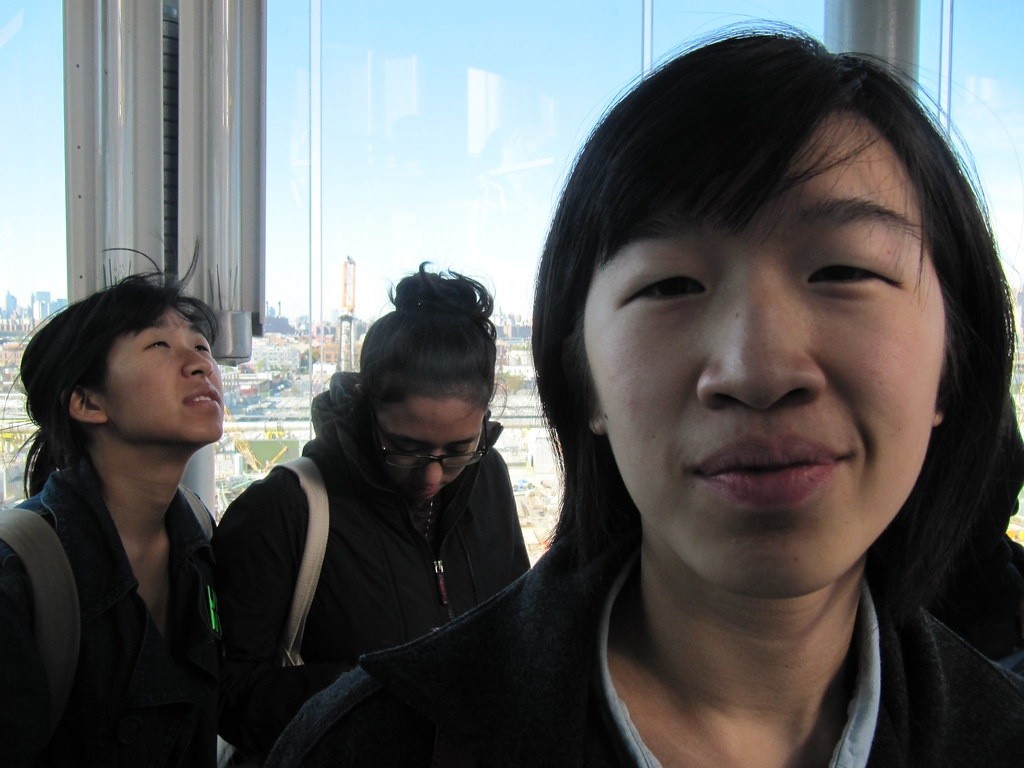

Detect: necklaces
[421,498,437,543]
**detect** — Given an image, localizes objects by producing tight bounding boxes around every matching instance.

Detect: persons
[259,18,1024,768]
[209,259,533,768]
[0,237,223,768]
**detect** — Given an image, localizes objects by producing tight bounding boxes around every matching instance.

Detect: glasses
[365,399,490,470]
